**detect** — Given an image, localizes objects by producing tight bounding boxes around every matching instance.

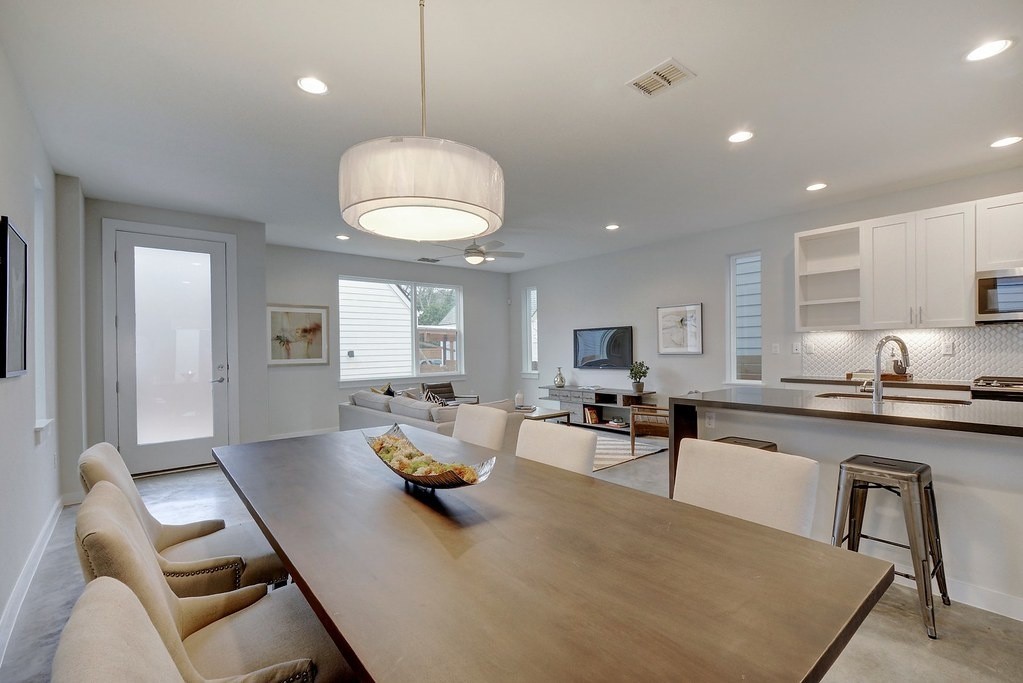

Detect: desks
[211,422,894,683]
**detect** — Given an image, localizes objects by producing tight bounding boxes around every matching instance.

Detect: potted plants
[629,361,649,394]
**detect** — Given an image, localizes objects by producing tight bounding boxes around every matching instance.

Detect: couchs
[338,391,524,454]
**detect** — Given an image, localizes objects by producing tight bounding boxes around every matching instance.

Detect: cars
[419,359,442,366]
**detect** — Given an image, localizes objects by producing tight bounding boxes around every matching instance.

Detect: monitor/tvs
[574,326,633,371]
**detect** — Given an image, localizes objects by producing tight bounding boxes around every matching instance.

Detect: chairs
[52,577,184,683]
[77,442,289,588]
[673,437,818,539]
[452,404,507,451]
[630,390,700,456]
[77,480,352,682]
[422,381,479,406]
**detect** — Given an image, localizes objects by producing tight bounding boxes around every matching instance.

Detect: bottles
[886,348,899,373]
[515,393,523,405]
[554,367,566,387]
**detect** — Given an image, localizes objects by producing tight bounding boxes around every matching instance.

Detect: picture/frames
[0,215,27,377]
[266,303,330,368]
[657,303,703,356]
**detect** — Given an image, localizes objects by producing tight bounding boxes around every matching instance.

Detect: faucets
[859,334,910,404]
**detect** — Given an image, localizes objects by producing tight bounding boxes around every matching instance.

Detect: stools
[711,436,778,453]
[832,455,951,641]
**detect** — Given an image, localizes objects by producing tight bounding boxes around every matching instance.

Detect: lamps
[337,1,504,244]
[463,238,485,266]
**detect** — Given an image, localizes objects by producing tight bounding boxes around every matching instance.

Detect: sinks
[814,392,973,405]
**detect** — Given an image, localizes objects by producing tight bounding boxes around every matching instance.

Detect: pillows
[370,382,417,400]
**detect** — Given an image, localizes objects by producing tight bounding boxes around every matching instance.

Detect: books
[515,405,536,413]
[606,416,628,428]
[585,407,599,423]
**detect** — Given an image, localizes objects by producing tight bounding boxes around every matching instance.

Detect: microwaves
[975,267,1023,324]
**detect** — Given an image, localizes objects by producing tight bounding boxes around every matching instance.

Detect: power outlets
[793,342,801,353]
[942,342,953,355]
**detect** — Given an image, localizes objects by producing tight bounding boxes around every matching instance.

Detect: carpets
[591,436,669,472]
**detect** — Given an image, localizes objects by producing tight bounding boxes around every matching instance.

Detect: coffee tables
[507,404,570,429]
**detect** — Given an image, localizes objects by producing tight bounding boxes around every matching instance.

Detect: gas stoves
[970,376,1023,401]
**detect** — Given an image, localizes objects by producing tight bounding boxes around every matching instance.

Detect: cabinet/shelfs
[538,385,656,436]
[794,192,1023,336]
